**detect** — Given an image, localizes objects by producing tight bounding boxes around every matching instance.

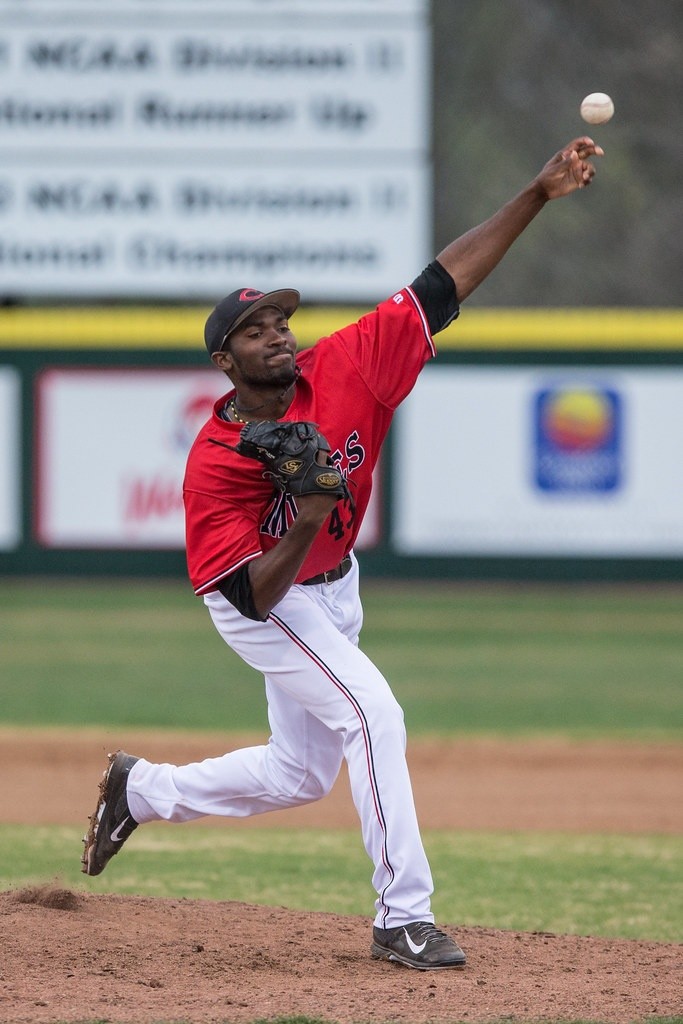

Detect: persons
[82,138,605,969]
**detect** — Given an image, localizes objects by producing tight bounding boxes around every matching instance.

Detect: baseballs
[580,92,615,126]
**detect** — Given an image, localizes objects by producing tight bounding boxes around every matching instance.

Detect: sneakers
[370,921,467,969]
[81,750,141,876]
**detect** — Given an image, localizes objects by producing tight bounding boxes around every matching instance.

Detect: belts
[299,554,352,586]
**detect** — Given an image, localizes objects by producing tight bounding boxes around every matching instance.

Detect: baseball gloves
[207,418,356,508]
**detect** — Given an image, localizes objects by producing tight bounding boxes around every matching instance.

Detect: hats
[204,288,300,356]
